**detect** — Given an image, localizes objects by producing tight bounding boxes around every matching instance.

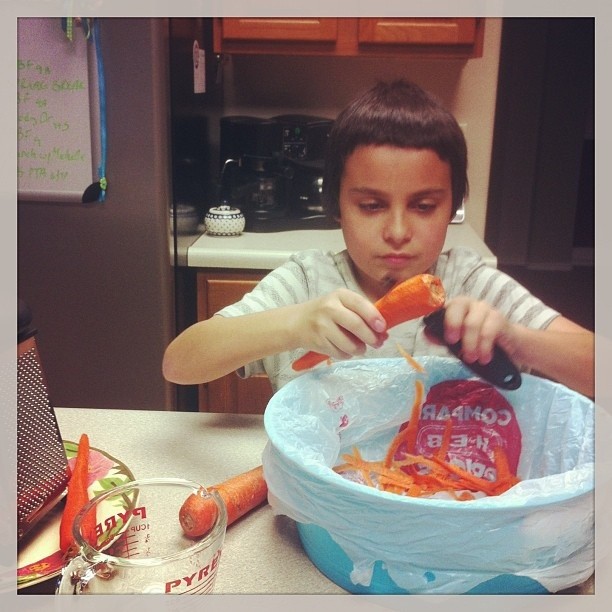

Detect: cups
[73,479,226,595]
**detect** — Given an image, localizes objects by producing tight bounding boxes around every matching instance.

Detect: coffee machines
[218,120,331,234]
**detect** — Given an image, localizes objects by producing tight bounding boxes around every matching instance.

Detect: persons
[161,79,594,402]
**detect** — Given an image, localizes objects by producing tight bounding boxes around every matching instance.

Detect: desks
[55,404,347,592]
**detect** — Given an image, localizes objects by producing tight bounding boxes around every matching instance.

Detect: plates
[17,441,138,590]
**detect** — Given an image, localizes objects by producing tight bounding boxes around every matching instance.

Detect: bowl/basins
[263,355,597,594]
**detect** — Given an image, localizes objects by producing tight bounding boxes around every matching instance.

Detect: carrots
[291,275,446,371]
[59,434,97,554]
[178,465,268,539]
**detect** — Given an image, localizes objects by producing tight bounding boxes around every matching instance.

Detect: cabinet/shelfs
[195,269,272,414]
[212,17,486,60]
